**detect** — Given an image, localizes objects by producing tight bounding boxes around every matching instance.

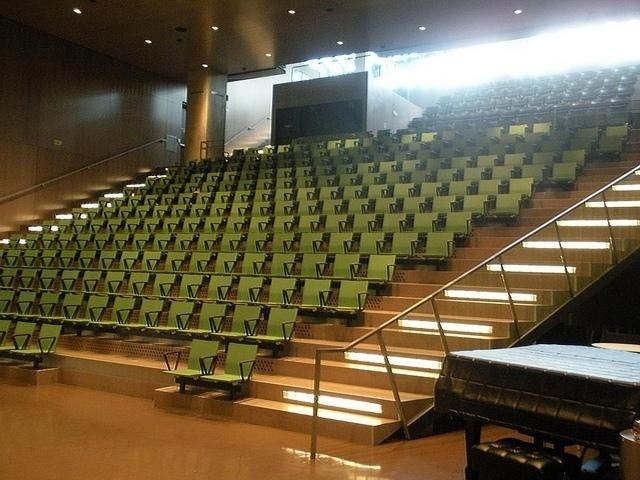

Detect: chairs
[1,131,640,320]
[178,303,226,337]
[88,297,136,334]
[10,323,63,368]
[200,343,259,398]
[161,339,218,393]
[209,305,262,340]
[247,306,300,356]
[64,297,110,336]
[0,321,38,359]
[116,299,164,335]
[404,58,638,131]
[146,301,195,336]
[0,321,10,346]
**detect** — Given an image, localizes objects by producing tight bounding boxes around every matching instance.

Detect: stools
[467,438,581,478]
